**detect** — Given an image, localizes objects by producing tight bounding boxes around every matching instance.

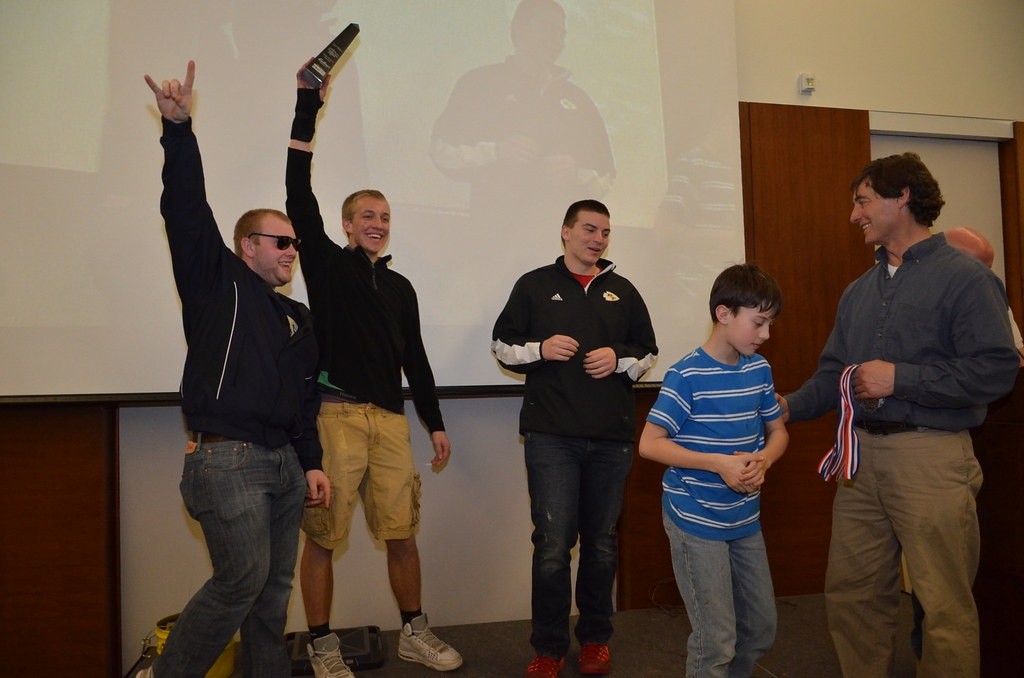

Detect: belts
[854,419,918,437]
[192,431,236,443]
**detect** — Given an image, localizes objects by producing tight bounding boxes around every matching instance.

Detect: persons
[428,0,616,305]
[138,61,332,678]
[639,263,788,678]
[285,57,462,678]
[490,200,659,678]
[773,153,1024,678]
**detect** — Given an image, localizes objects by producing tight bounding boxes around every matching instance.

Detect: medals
[858,397,885,414]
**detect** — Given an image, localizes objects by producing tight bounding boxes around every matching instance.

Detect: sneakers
[397,613,464,671]
[525,655,565,678]
[577,641,612,675]
[306,633,355,678]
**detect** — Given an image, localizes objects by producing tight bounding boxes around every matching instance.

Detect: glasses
[248,233,302,252]
